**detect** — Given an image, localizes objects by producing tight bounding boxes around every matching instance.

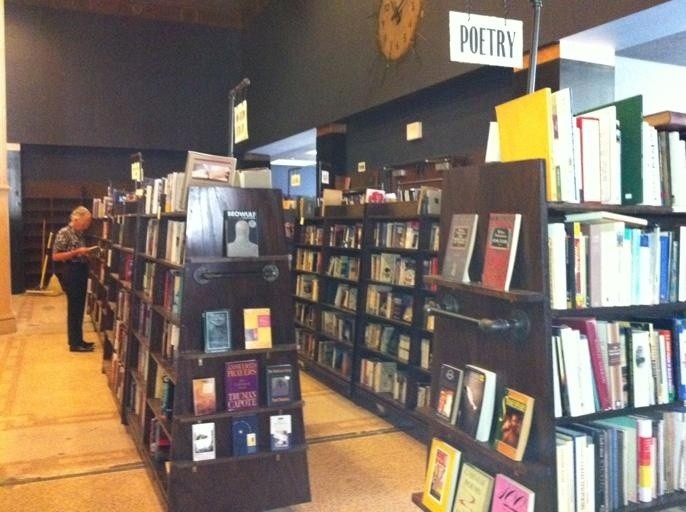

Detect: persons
[500,413,521,448]
[436,389,447,415]
[50,205,96,352]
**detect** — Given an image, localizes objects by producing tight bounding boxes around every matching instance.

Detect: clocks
[371,0,425,67]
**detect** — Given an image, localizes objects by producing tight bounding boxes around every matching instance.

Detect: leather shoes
[71,344,93,351]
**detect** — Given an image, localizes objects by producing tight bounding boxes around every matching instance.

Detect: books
[280,185,445,410]
[486,86,686,211]
[191,414,292,460]
[422,437,536,512]
[551,315,686,416]
[192,360,293,417]
[86,219,184,474]
[556,412,684,512]
[549,210,685,310]
[91,151,272,216]
[203,307,271,352]
[441,213,520,291]
[225,209,259,257]
[434,363,534,463]
